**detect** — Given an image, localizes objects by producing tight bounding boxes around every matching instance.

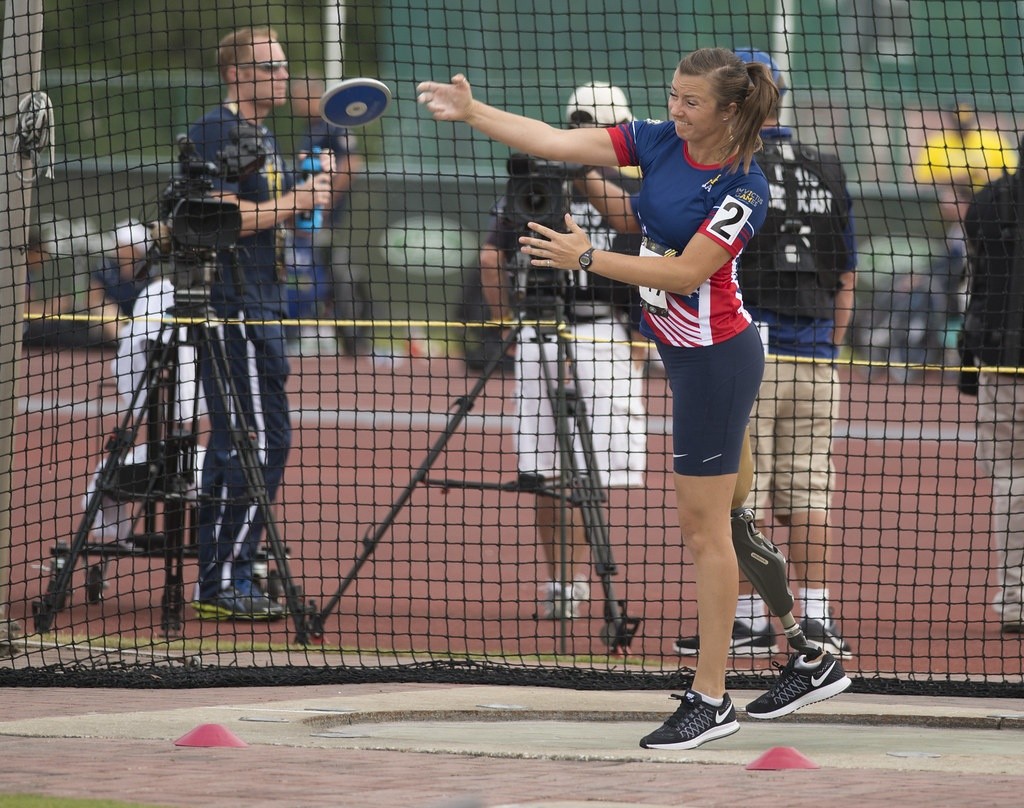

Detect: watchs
[579,248,596,271]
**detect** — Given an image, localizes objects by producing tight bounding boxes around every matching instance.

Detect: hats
[735,49,787,94]
[566,83,639,125]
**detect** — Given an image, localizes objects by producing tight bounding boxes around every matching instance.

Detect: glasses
[238,61,288,74]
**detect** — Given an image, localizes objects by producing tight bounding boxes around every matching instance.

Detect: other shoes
[1001,624,1024,633]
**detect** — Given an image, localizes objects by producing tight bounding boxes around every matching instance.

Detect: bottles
[292,145,323,232]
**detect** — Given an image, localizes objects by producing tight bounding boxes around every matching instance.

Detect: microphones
[505,153,534,176]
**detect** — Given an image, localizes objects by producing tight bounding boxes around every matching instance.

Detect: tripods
[30,255,310,643]
[313,290,635,646]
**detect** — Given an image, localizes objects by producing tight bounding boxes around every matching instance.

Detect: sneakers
[188,585,290,621]
[672,620,780,659]
[746,651,851,719]
[544,577,589,620]
[640,689,741,751]
[787,618,852,661]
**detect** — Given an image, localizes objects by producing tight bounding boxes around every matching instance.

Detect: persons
[479,81,651,622]
[164,27,335,622]
[415,45,852,752]
[917,99,1023,636]
[673,48,857,660]
[108,71,383,361]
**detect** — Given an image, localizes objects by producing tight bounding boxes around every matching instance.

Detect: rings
[547,259,550,268]
